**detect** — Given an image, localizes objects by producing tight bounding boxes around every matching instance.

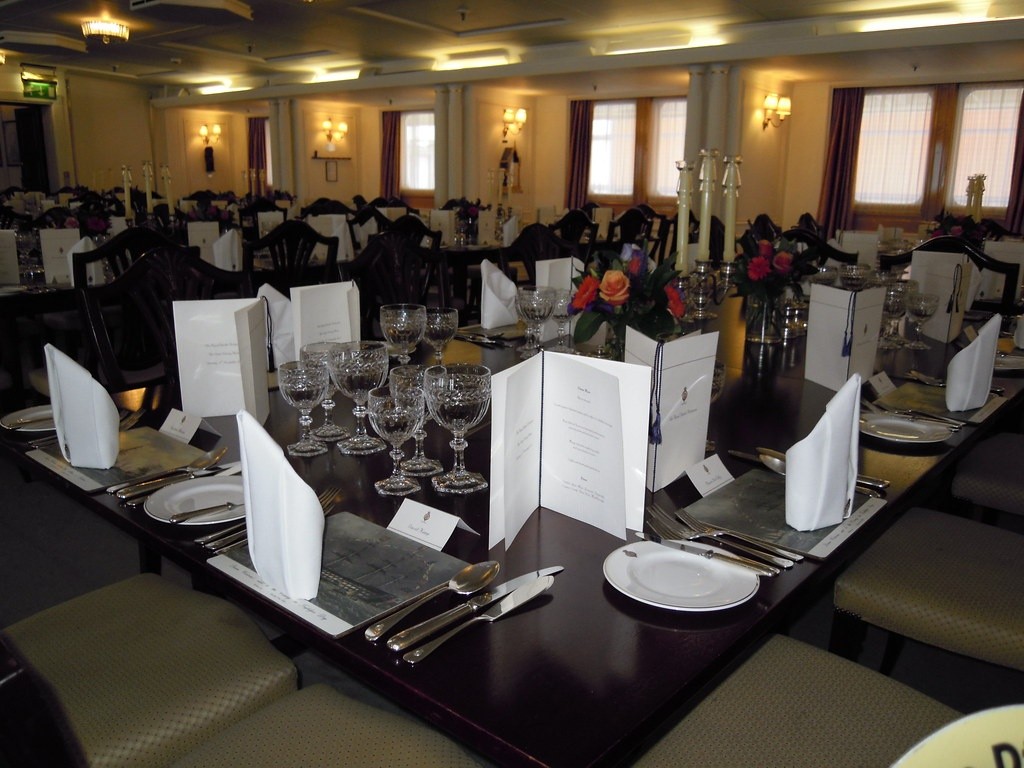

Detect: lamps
[200,122,221,145]
[761,92,793,131]
[322,118,349,142]
[81,18,131,47]
[501,107,526,136]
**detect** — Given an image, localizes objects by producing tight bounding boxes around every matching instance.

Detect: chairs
[0,183,1024,768]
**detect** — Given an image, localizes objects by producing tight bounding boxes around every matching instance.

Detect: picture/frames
[325,160,338,183]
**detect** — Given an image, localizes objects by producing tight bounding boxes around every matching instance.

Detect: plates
[992,354,1024,371]
[0,404,56,432]
[858,411,952,442]
[145,475,247,525]
[602,536,760,610]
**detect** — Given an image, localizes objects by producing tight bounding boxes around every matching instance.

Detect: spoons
[760,453,882,501]
[911,370,1000,398]
[364,560,499,641]
[105,445,228,491]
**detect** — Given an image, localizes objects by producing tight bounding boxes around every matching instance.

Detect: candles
[675,158,694,277]
[141,159,157,215]
[159,160,176,215]
[966,173,988,222]
[696,146,719,262]
[121,165,133,221]
[487,167,512,207]
[241,167,266,198]
[721,154,743,262]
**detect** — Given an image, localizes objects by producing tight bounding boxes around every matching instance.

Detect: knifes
[385,565,565,650]
[891,372,1005,394]
[403,573,554,663]
[117,459,243,507]
[727,446,889,492]
[634,531,777,578]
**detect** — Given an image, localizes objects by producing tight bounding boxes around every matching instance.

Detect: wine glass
[7,220,116,289]
[452,208,524,247]
[276,302,487,499]
[812,262,934,352]
[514,286,611,359]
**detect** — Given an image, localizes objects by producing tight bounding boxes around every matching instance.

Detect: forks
[643,503,804,572]
[28,409,142,449]
[195,483,343,554]
[862,398,966,431]
